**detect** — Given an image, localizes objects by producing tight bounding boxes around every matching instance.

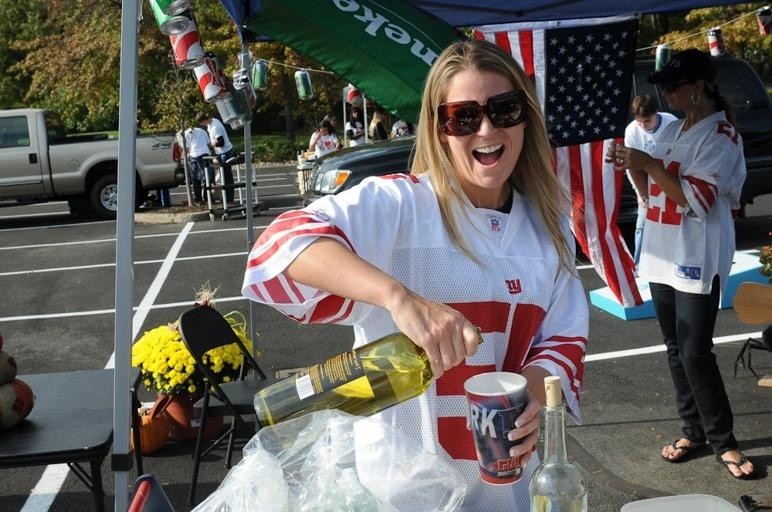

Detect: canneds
[294,70,314,101]
[149,0,268,130]
[347,83,362,104]
[756,6,772,36]
[655,44,672,72]
[706,28,726,57]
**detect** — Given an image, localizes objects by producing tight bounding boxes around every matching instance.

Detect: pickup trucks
[0,107,182,218]
[304,56,772,236]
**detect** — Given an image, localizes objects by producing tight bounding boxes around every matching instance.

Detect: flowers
[130,312,264,396]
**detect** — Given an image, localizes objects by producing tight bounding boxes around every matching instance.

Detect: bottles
[253,326,485,452]
[527,375,590,511]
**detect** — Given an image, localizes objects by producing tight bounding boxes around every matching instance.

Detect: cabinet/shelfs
[194,146,261,221]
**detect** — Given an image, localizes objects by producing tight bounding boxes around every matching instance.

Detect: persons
[622,93,680,268]
[240,39,589,512]
[605,48,757,481]
[308,108,417,152]
[174,111,234,204]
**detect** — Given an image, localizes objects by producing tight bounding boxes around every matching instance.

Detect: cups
[464,371,528,486]
[298,155,305,164]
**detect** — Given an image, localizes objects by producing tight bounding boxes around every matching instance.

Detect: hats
[647,49,716,84]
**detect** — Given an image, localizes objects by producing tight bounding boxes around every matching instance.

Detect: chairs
[175,303,288,508]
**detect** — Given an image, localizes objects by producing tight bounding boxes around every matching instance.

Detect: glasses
[437,90,526,136]
[660,83,687,93]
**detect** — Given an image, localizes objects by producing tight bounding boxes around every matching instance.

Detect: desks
[0,363,147,512]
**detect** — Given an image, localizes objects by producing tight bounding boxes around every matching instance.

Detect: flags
[470,15,647,308]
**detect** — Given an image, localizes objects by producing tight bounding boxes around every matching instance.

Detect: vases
[157,391,227,442]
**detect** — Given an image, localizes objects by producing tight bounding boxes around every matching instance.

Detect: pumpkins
[131,408,168,456]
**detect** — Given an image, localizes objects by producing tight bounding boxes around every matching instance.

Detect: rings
[621,159,624,164]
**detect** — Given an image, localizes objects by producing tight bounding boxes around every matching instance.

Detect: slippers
[716,452,760,479]
[661,438,704,462]
